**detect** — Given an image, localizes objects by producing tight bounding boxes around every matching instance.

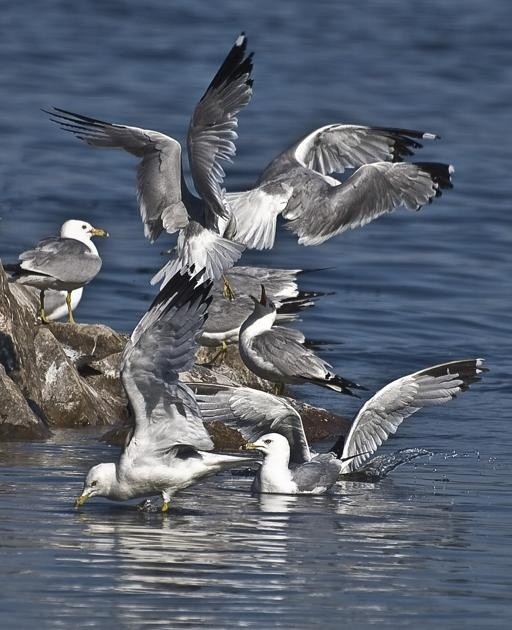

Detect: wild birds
[71,264,489,513]
[259,122,457,248]
[5,220,109,322]
[39,27,264,282]
